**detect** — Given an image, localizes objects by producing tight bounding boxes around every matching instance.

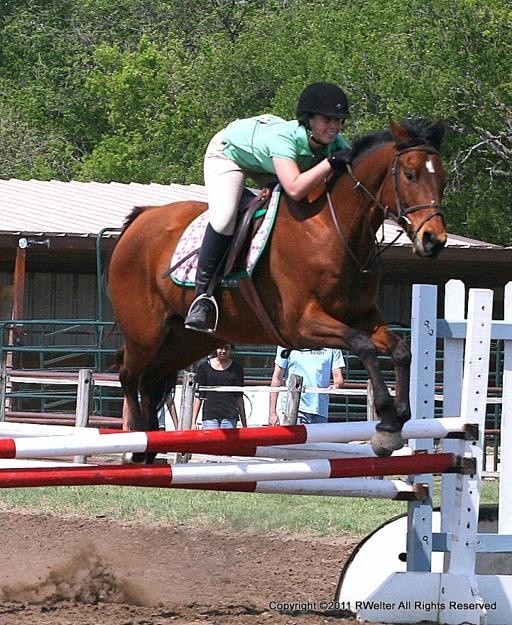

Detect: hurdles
[1,271,511,624]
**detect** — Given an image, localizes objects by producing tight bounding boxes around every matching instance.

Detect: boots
[184,222,233,330]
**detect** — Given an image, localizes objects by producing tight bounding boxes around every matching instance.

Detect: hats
[296,82,352,120]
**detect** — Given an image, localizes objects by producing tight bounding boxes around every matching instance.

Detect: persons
[184,81,352,327]
[191,343,247,431]
[269,346,346,426]
[122,391,178,431]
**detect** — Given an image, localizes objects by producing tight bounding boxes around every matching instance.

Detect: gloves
[328,151,353,173]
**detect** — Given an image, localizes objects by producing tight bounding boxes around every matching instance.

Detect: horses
[101,110,451,466]
